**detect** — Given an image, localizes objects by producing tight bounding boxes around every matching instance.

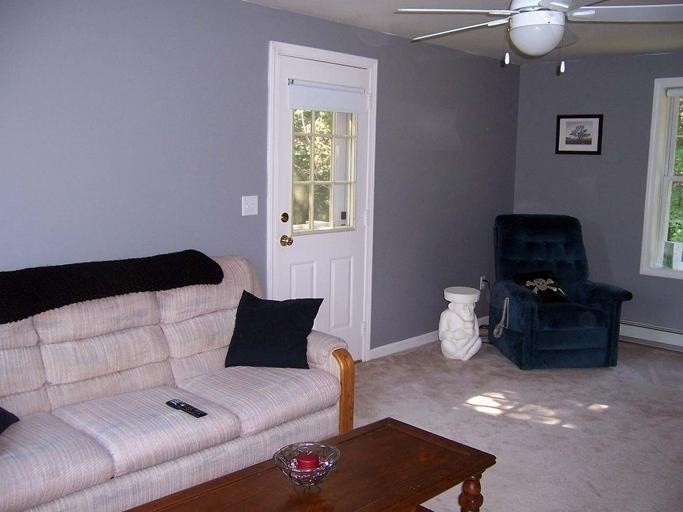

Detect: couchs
[1,255,355,512]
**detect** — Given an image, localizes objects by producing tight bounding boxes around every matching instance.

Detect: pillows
[225,290,323,369]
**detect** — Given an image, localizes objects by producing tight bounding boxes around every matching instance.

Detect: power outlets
[480,276,487,290]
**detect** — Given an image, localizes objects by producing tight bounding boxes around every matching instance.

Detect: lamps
[509,11,565,56]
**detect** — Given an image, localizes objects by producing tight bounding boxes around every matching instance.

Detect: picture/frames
[555,113,603,155]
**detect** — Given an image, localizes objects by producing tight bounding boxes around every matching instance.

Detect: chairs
[489,213,632,370]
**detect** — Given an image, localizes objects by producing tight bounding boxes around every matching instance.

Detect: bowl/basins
[270,440,343,487]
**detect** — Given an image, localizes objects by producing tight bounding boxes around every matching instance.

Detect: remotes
[168,399,207,417]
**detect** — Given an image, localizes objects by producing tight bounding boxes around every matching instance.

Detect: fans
[394,1,683,56]
